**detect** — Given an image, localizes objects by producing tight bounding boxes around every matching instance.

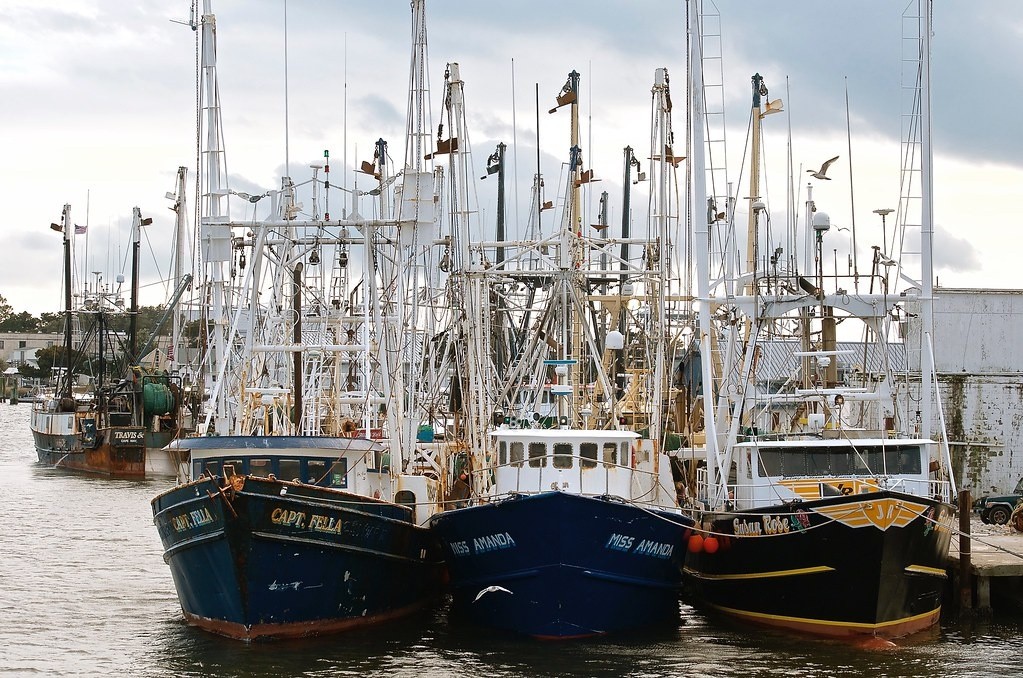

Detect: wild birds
[472,585,512,603]
[878,252,903,268]
[830,224,850,232]
[806,155,839,180]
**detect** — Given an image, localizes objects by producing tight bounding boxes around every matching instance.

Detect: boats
[148,2,466,648]
[679,1,960,651]
[428,4,696,649]
[28,205,186,480]
[18,386,54,403]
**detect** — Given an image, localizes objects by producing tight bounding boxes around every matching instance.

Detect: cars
[974,477,1023,525]
